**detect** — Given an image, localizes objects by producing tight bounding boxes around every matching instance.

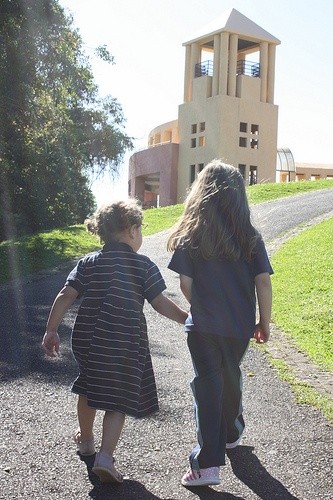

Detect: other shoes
[180,467,220,487]
[92,452,123,484]
[225,434,241,449]
[72,427,95,456]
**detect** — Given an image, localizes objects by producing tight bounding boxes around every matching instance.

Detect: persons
[167,161,275,487]
[42,200,189,484]
